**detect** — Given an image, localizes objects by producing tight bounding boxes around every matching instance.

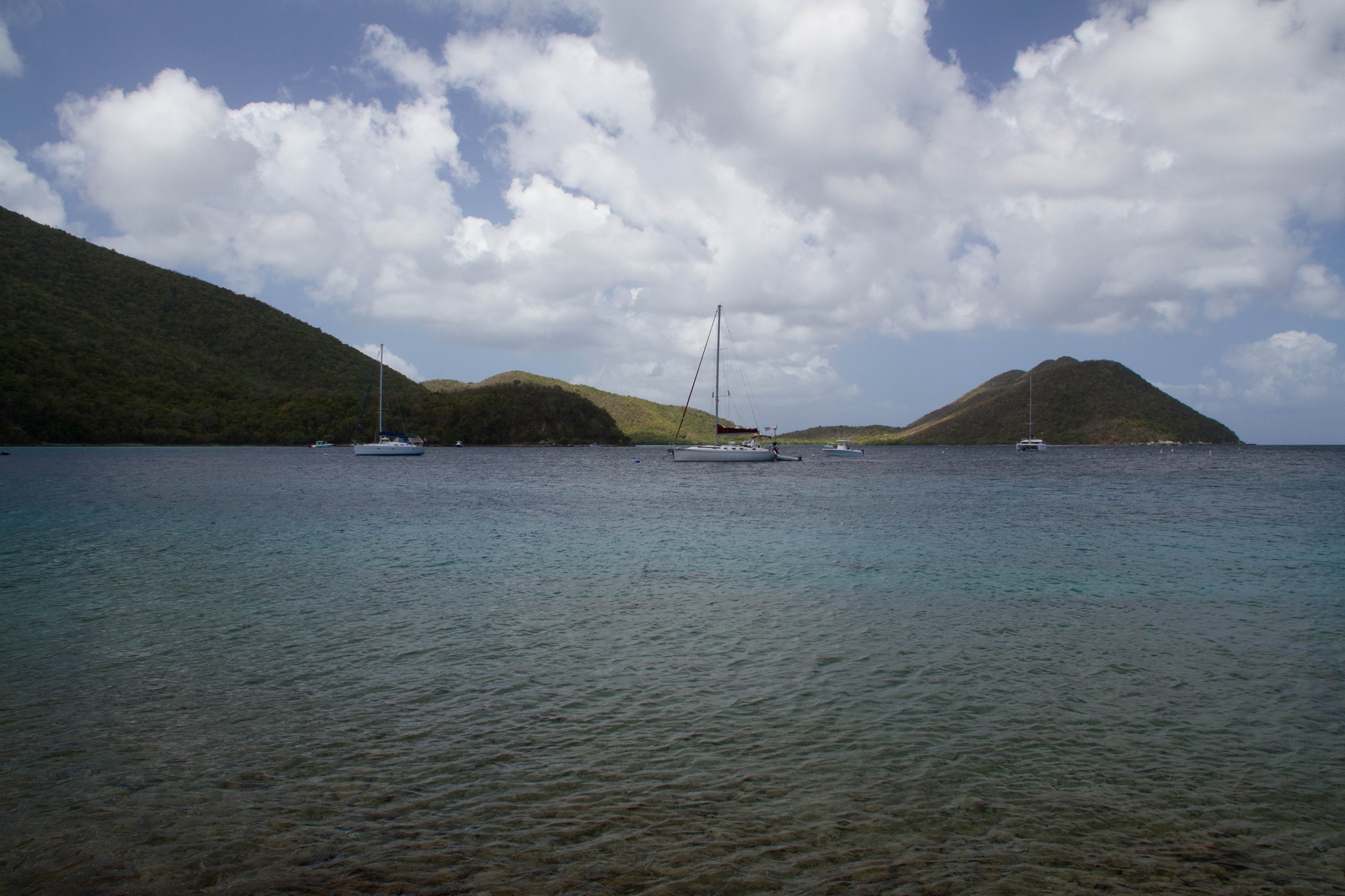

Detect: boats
[308,439,334,448]
[821,442,851,449]
[822,424,867,457]
[455,437,464,447]
[773,449,803,461]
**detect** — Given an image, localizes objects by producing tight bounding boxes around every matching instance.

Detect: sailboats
[1015,375,1048,452]
[667,302,778,462]
[349,343,425,456]
